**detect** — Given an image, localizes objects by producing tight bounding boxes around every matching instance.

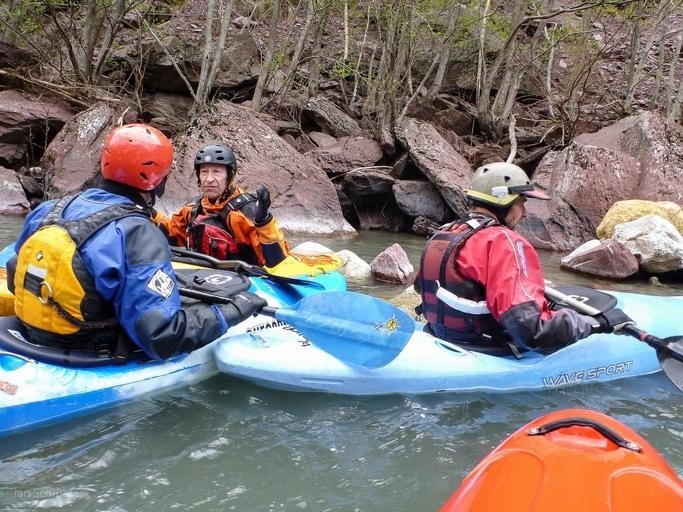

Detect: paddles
[1,240,324,290]
[543,284,683,391]
[178,286,415,369]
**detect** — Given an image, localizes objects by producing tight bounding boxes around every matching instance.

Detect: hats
[515,189,551,200]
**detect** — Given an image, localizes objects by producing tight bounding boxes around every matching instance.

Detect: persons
[145,140,290,268]
[6,123,267,360]
[412,161,635,359]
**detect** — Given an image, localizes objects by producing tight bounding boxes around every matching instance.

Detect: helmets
[101,122,173,198]
[194,143,237,187]
[466,161,552,207]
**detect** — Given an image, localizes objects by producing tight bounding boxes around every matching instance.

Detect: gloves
[252,184,272,223]
[592,307,634,336]
[220,291,268,326]
